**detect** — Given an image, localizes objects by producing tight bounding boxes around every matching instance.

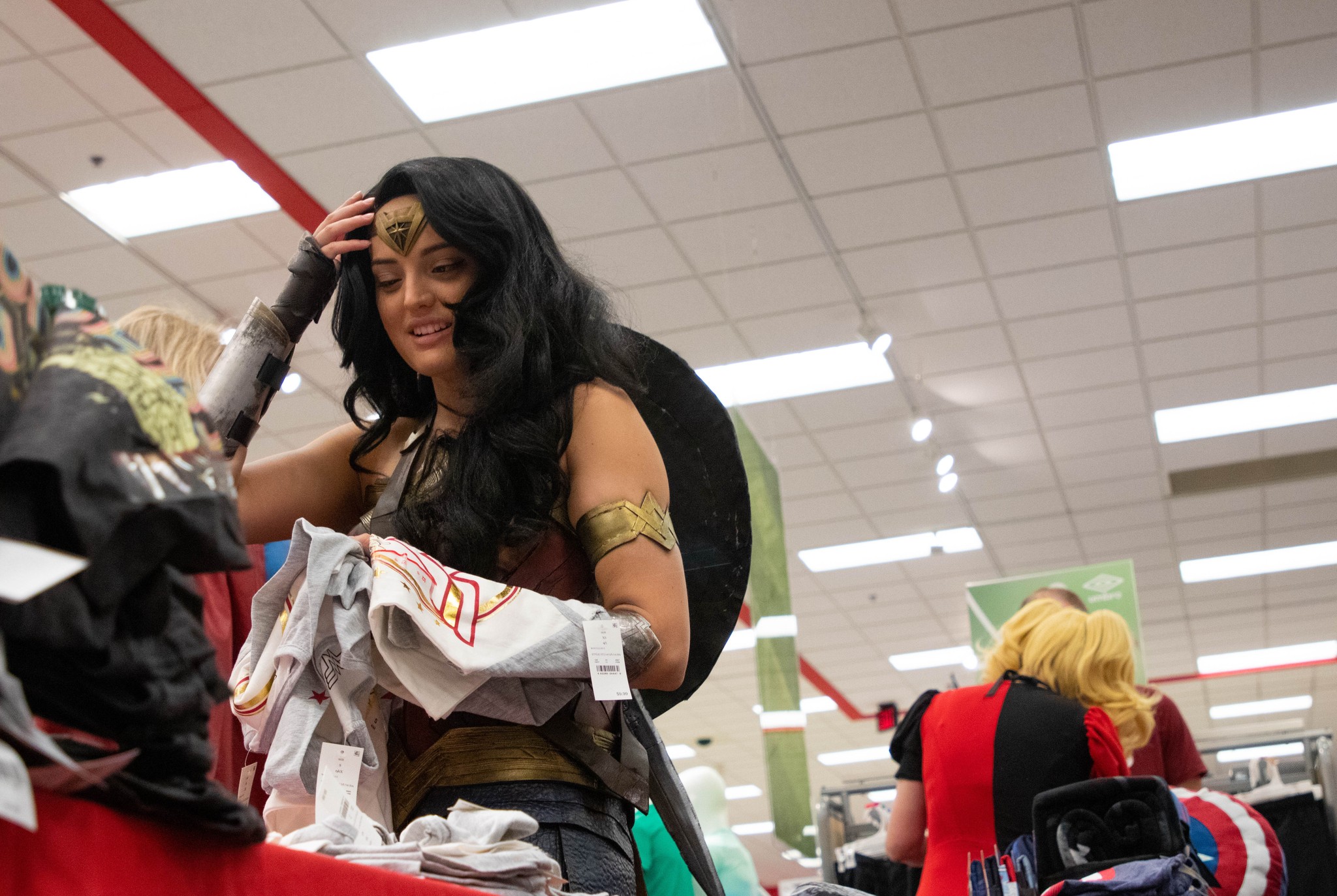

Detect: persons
[191,156,690,896]
[885,585,1207,896]
[104,306,280,824]
